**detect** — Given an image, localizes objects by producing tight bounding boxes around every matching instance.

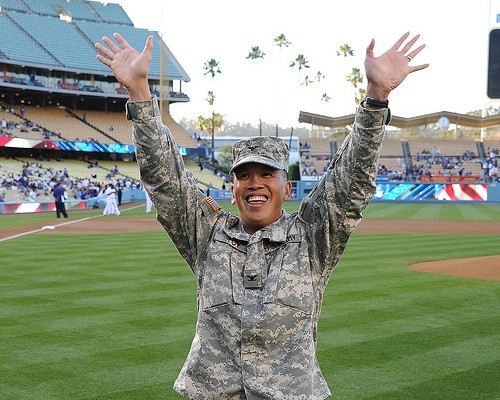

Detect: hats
[229,136,291,175]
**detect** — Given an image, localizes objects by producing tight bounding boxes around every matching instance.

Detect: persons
[111,124,114,131]
[413,147,500,169]
[0,105,51,139]
[299,142,311,158]
[103,183,122,216]
[94,32,429,400]
[53,183,69,218]
[0,162,143,203]
[377,164,500,182]
[222,183,225,189]
[300,161,330,176]
[83,113,86,121]
[95,160,98,168]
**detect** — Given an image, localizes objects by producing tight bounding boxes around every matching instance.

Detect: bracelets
[366,96,389,109]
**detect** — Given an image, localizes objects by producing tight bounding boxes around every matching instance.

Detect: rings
[405,55,412,61]
